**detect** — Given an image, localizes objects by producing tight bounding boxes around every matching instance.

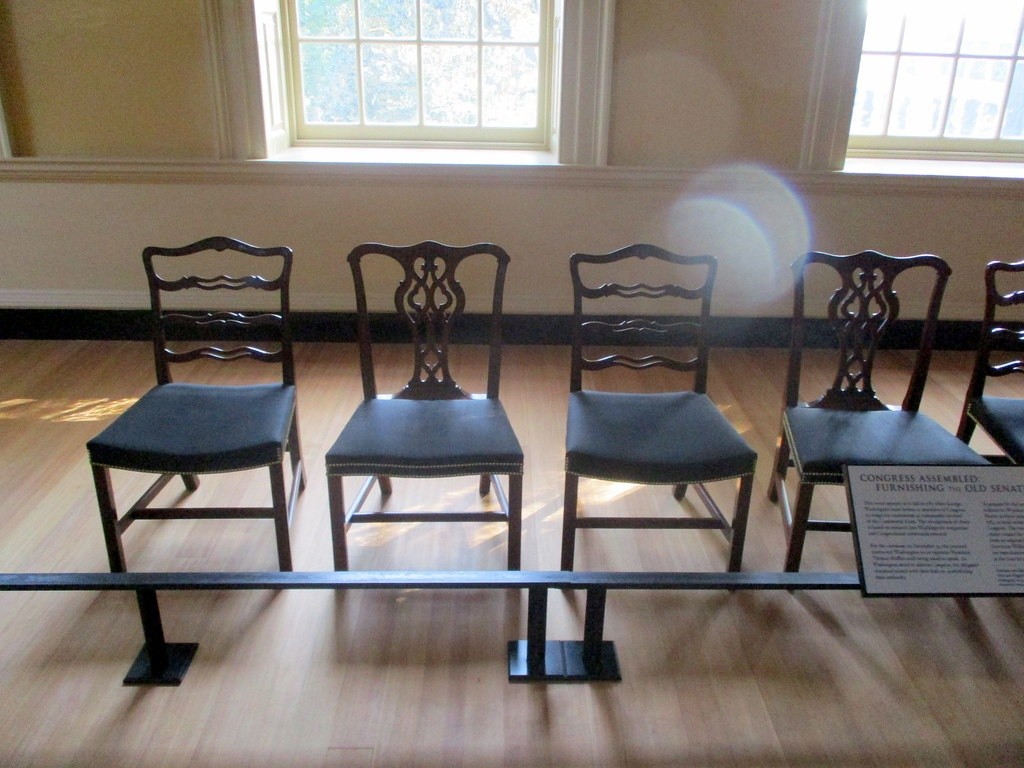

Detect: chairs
[85,235,308,573]
[560,243,759,570]
[324,238,526,568]
[957,259,1024,468]
[766,250,993,572]
